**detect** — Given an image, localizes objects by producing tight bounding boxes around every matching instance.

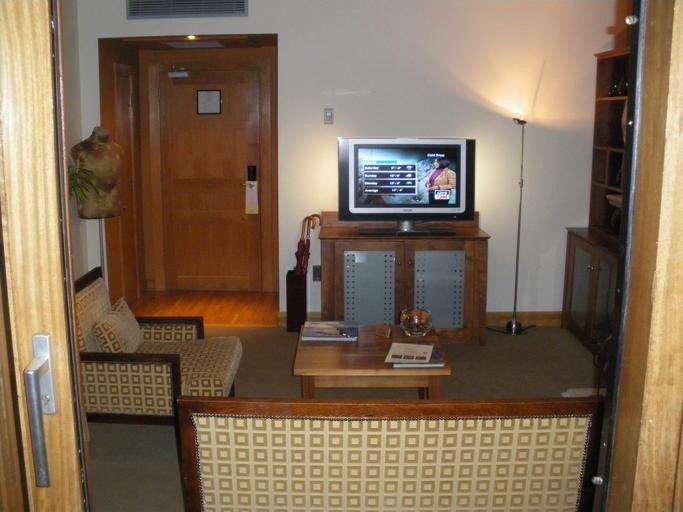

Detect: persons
[70,126,125,219]
[425,157,456,205]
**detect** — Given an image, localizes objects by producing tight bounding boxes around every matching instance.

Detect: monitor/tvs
[339,137,475,237]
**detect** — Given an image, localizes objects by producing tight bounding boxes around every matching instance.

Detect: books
[384,342,445,368]
[302,321,358,341]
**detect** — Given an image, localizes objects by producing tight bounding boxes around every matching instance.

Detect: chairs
[72,266,241,426]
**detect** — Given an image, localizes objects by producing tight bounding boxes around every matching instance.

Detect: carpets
[77,325,606,511]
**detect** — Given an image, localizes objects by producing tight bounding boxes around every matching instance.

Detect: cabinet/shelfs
[317,212,492,330]
[560,48,639,372]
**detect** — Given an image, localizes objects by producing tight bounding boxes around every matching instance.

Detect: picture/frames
[195,88,222,115]
